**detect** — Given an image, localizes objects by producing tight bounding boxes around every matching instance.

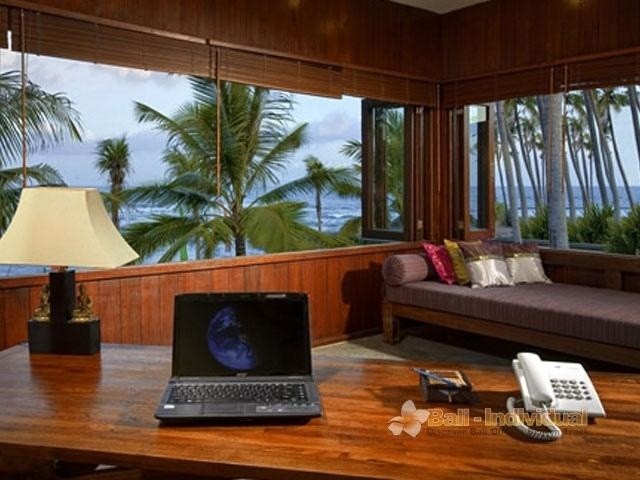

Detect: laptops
[153,291,324,424]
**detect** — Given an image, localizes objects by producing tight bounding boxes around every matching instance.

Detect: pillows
[421,237,554,289]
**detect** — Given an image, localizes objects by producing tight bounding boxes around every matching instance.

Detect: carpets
[311,334,511,363]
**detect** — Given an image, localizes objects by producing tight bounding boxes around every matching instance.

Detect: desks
[0,340,640,480]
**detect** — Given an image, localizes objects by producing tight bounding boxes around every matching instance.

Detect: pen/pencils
[413,367,460,389]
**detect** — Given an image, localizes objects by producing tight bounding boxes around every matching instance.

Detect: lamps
[0,187,141,355]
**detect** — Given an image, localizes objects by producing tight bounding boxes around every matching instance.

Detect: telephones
[511,352,607,415]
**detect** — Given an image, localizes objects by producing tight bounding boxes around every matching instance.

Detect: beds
[380,247,640,368]
